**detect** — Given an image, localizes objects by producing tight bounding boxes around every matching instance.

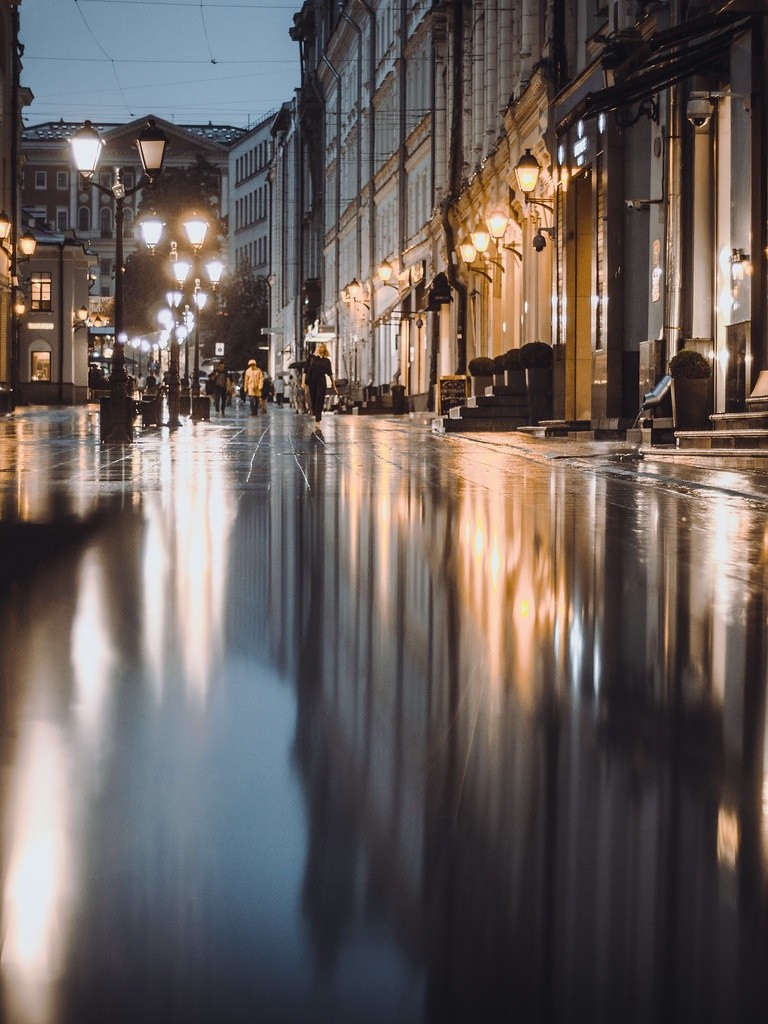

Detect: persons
[208,360,231,416]
[301,342,339,430]
[145,370,157,388]
[204,371,296,409]
[244,359,264,417]
[136,372,146,400]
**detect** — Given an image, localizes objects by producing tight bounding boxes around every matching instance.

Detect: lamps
[337,260,394,303]
[459,139,542,266]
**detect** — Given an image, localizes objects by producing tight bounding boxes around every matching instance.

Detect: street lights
[67,118,169,447]
[135,249,227,420]
[139,205,212,433]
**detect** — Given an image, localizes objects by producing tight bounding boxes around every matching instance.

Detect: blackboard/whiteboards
[437,375,468,416]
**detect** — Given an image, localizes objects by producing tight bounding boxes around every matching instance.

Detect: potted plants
[466,342,552,396]
[667,350,712,430]
